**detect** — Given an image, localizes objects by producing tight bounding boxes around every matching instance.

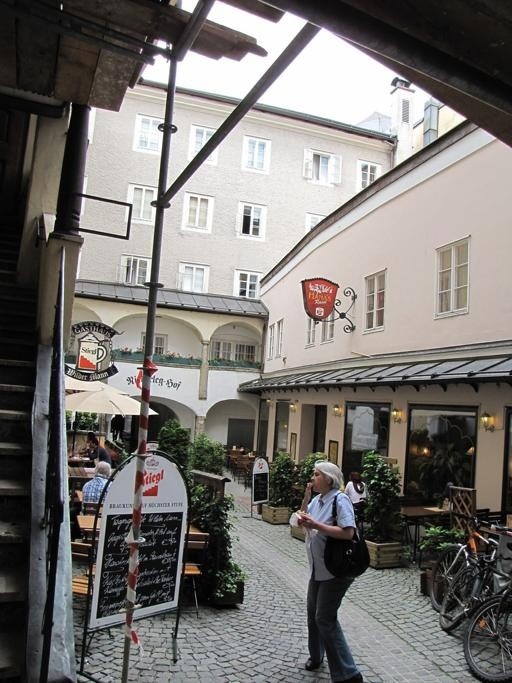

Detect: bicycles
[430,511,512,683]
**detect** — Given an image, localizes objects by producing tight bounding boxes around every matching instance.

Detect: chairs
[226,448,277,492]
[419,508,507,570]
[67,440,210,638]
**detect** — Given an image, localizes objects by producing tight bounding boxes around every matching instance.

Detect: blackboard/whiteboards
[84,448,191,633]
[251,456,269,504]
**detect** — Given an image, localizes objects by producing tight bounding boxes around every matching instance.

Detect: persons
[110,414,125,441]
[79,459,114,510]
[86,437,113,467]
[80,432,99,455]
[341,471,370,522]
[294,460,367,682]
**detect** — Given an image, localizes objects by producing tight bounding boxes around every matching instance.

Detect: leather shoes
[305,658,324,671]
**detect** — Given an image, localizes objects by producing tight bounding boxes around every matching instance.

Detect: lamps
[289,399,495,433]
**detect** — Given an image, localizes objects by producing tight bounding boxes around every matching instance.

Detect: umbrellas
[64,379,130,396]
[65,373,89,385]
[64,385,160,441]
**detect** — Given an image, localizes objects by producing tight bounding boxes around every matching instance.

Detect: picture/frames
[329,440,338,465]
[290,433,296,460]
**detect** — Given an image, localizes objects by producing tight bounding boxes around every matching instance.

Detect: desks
[400,506,450,564]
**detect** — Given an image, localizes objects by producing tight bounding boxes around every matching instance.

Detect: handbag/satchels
[324,492,370,577]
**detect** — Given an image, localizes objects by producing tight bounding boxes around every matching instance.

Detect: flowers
[120,344,193,361]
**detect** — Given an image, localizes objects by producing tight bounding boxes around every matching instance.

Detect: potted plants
[421,526,466,602]
[262,451,330,542]
[361,450,406,569]
